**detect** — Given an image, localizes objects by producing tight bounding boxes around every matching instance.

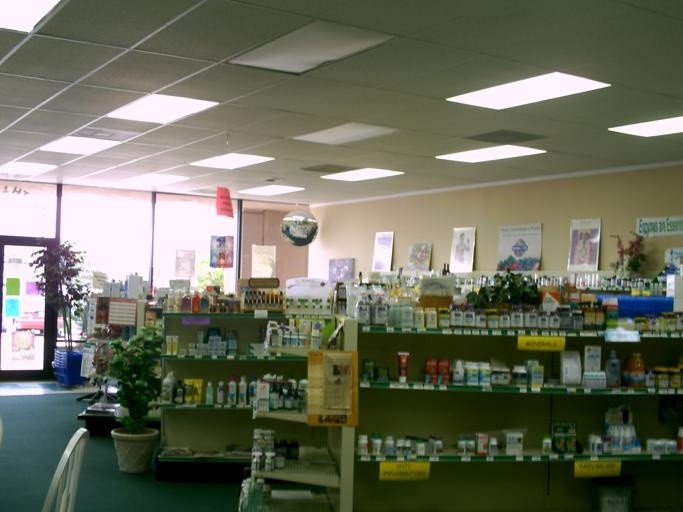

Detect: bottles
[604,350,683,389]
[453,360,527,387]
[162,371,203,404]
[457,274,494,287]
[570,267,577,289]
[576,273,601,289]
[358,432,500,457]
[438,301,607,329]
[534,272,569,287]
[237,478,270,511]
[631,277,664,297]
[259,320,320,349]
[250,426,300,472]
[354,291,438,329]
[602,274,632,293]
[635,310,683,336]
[204,373,307,411]
[167,284,240,314]
[541,431,603,456]
[241,277,333,316]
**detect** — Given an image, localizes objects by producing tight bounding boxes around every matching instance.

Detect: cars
[11,311,70,337]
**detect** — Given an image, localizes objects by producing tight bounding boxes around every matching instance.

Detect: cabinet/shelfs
[154,311,329,471]
[239,319,344,511]
[96,297,148,386]
[339,320,682,512]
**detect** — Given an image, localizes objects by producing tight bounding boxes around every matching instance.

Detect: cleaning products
[606,350,621,387]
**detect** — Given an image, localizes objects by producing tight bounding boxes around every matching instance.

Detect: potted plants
[108,327,163,474]
[467,266,539,315]
[29,236,92,388]
